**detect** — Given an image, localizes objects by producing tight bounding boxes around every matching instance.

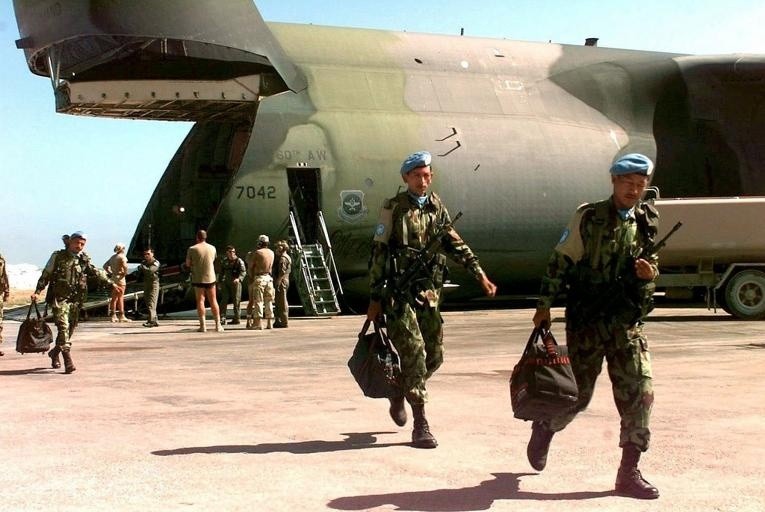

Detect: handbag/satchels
[348,332,403,399]
[16,318,53,353]
[510,344,577,421]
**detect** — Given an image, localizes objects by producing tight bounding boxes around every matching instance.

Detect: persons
[31,232,123,374]
[0,254,9,356]
[139,248,161,326]
[527,154,660,499]
[367,151,497,448]
[103,245,130,322]
[187,229,291,331]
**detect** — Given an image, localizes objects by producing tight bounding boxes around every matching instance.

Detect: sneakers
[142,321,158,327]
[198,320,240,332]
[111,315,132,323]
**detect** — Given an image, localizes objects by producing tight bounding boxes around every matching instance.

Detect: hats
[257,234,269,242]
[400,151,430,173]
[71,231,86,240]
[113,244,123,252]
[609,153,654,176]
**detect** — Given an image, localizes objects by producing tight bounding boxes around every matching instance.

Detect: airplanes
[3,1,763,322]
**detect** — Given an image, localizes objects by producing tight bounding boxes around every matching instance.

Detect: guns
[577,220,682,323]
[43,264,60,317]
[391,211,464,307]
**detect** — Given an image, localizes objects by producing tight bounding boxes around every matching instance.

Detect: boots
[247,318,287,330]
[615,445,660,499]
[527,421,555,471]
[62,349,75,373]
[412,405,438,447]
[388,390,407,426]
[48,347,61,368]
